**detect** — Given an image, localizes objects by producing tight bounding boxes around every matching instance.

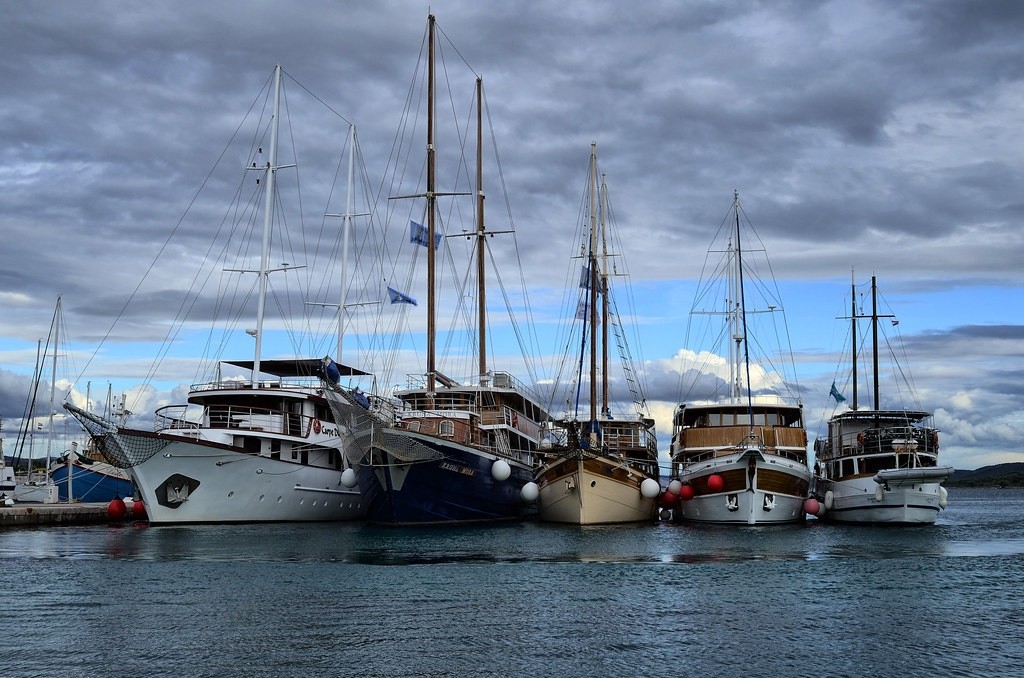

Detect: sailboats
[534,141,661,530]
[317,7,561,524]
[0,293,138,503]
[812,264,955,525]
[670,189,811,527]
[62,63,411,525]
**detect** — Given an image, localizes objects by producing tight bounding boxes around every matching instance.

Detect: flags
[387,286,417,306]
[411,220,442,250]
[830,381,846,403]
[578,266,604,298]
[574,302,601,328]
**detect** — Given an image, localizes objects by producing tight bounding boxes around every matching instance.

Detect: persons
[857,425,939,455]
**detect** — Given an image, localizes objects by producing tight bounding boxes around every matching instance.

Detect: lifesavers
[313,420,321,434]
